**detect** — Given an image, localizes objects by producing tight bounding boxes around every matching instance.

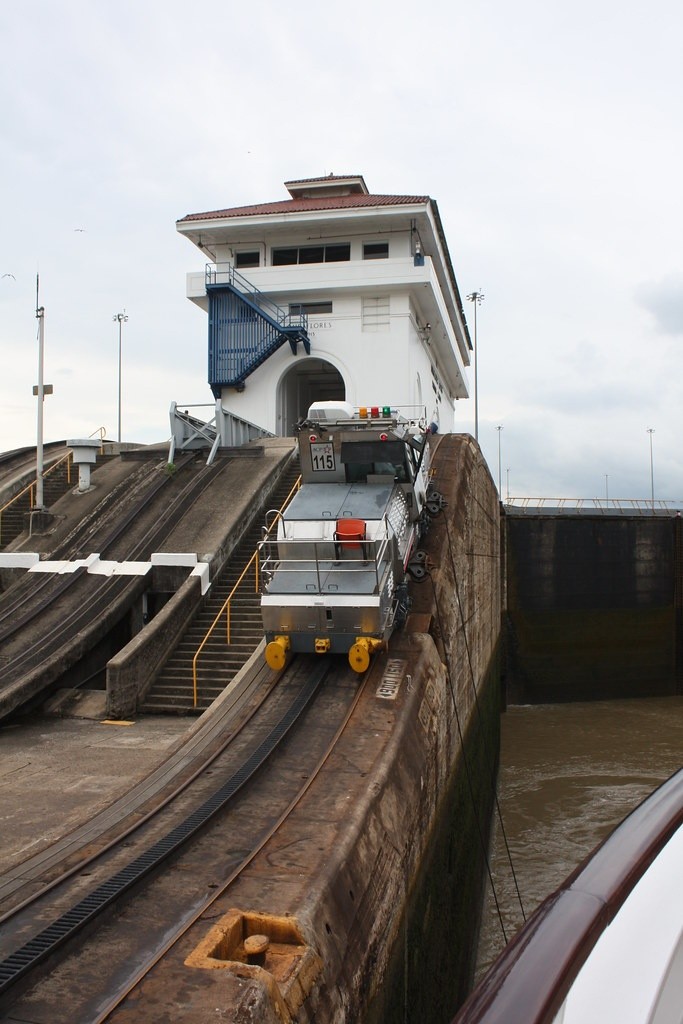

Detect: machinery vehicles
[259,400,448,674]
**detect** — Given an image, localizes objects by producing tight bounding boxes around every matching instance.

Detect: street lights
[467,290,484,439]
[495,425,504,503]
[647,427,656,509]
[506,467,510,504]
[113,313,130,442]
